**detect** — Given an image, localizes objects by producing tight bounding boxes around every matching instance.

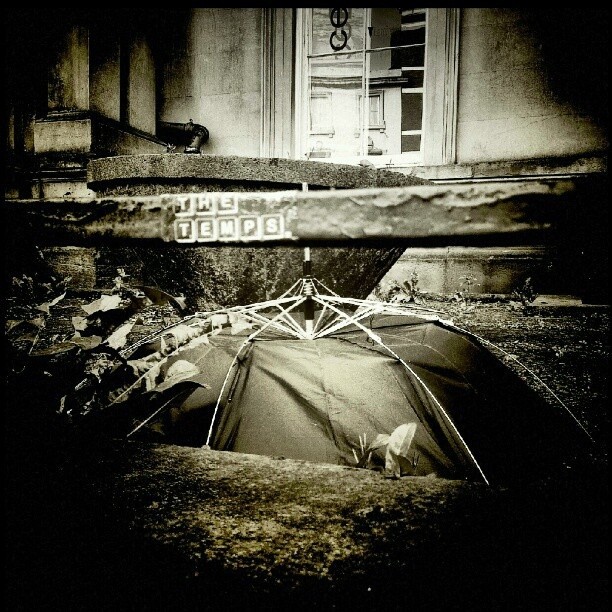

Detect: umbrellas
[63,245,601,487]
